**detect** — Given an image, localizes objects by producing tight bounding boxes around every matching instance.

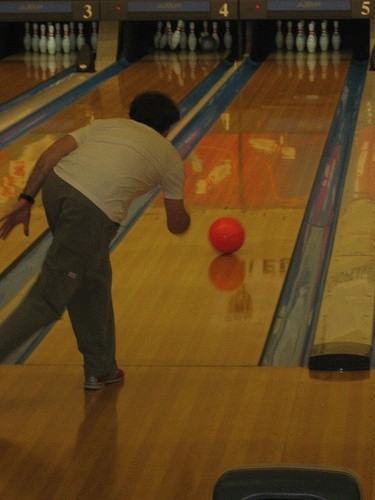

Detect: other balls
[208,217,245,254]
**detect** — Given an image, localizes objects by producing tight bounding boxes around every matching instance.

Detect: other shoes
[84,368,123,388]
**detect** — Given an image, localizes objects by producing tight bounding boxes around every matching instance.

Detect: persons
[0,89,191,390]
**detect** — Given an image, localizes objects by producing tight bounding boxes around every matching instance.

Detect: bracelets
[17,193,34,204]
